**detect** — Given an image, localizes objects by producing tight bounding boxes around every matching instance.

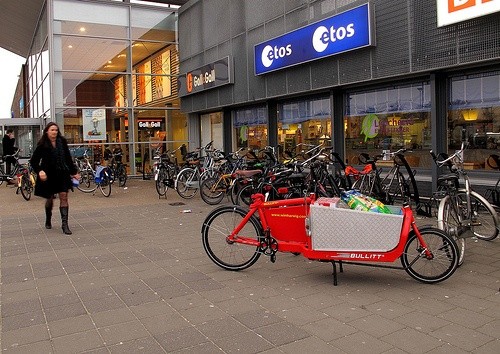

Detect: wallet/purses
[72,178,80,188]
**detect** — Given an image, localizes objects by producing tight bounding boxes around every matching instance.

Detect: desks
[451,163,481,170]
[376,159,395,167]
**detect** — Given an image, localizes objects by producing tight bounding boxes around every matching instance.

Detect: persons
[30,122,80,235]
[294,128,302,153]
[255,141,262,150]
[104,144,124,162]
[2,129,19,175]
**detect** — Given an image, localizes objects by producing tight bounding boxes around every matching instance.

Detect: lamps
[462,109,478,120]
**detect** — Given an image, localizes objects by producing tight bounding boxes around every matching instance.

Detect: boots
[60,206,72,235]
[45,202,53,229]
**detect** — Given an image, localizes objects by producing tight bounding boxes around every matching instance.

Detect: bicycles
[68,146,128,197]
[430,129,500,267]
[15,154,37,200]
[0,149,30,185]
[152,141,412,221]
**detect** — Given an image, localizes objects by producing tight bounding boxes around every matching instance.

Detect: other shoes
[6,182,16,187]
